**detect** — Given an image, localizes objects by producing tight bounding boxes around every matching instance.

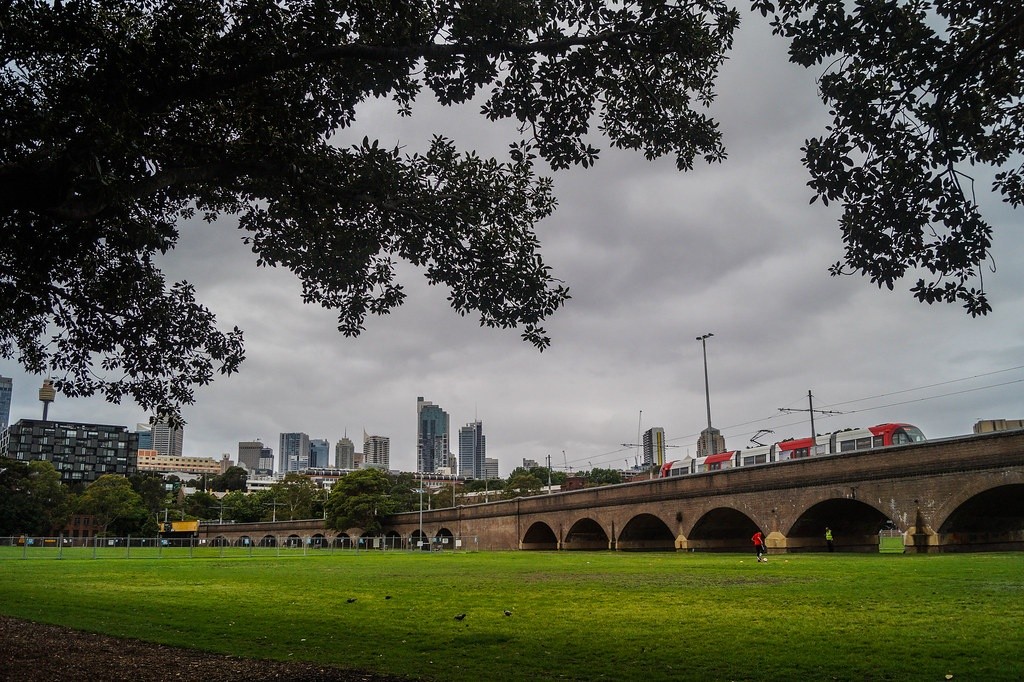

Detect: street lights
[417,442,425,551]
[695,332,715,455]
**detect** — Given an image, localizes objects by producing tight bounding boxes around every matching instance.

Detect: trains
[658,422,928,479]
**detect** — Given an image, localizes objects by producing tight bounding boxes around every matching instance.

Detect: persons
[824,527,834,552]
[751,530,765,562]
